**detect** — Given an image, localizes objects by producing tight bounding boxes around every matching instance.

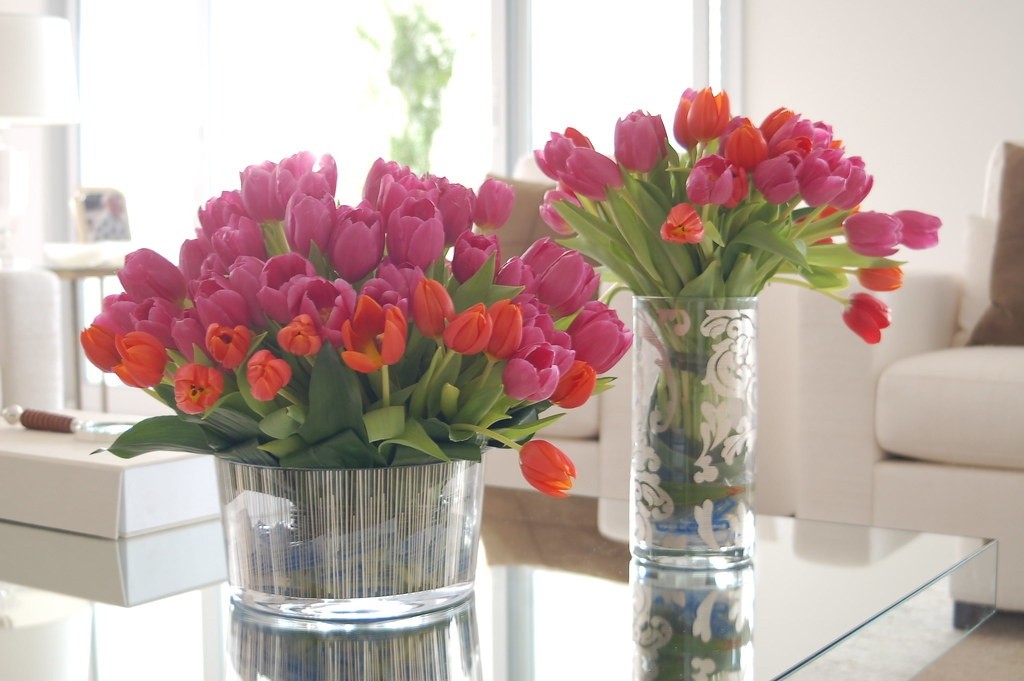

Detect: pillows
[960,140,1024,345]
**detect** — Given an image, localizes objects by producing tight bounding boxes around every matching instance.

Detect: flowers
[80,150,632,500]
[534,86,942,345]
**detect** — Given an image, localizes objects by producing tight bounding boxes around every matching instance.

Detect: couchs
[866,138,1022,630]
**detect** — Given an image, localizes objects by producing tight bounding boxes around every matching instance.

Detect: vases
[628,293,759,573]
[215,454,487,681]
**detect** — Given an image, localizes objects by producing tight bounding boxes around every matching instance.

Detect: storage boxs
[0,519,229,608]
[0,422,223,541]
